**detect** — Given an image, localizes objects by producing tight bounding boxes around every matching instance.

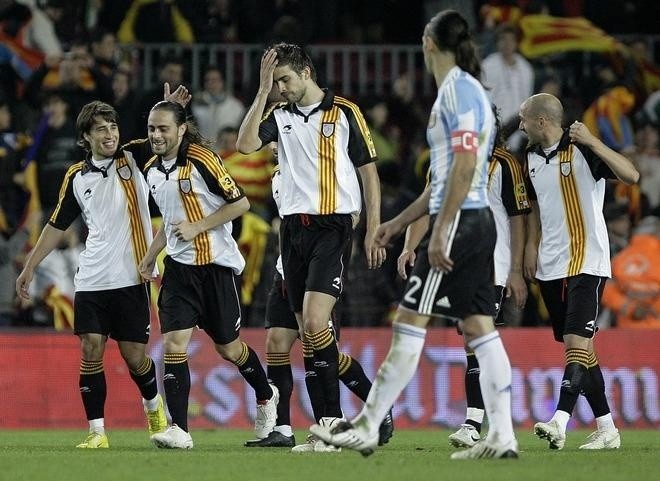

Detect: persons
[1,0,281,327]
[236,43,386,452]
[335,76,432,329]
[309,9,520,460]
[522,94,639,450]
[397,103,533,450]
[469,0,660,326]
[245,103,394,448]
[140,100,280,450]
[15,82,193,449]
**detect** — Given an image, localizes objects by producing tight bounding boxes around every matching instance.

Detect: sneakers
[76,432,109,449]
[579,428,621,449]
[534,420,566,449]
[144,395,193,449]
[292,404,394,457]
[449,423,481,448]
[450,432,518,459]
[244,431,295,447]
[255,384,281,439]
